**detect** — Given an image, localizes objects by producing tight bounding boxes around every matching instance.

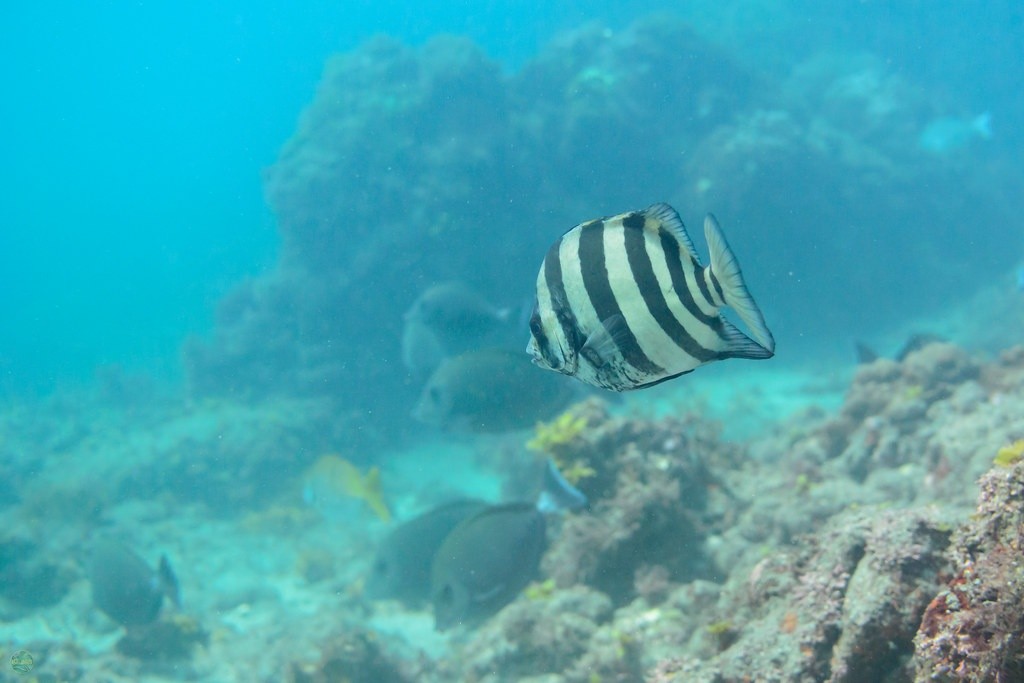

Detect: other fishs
[520,198,780,394]
[84,532,217,666]
[357,454,594,637]
